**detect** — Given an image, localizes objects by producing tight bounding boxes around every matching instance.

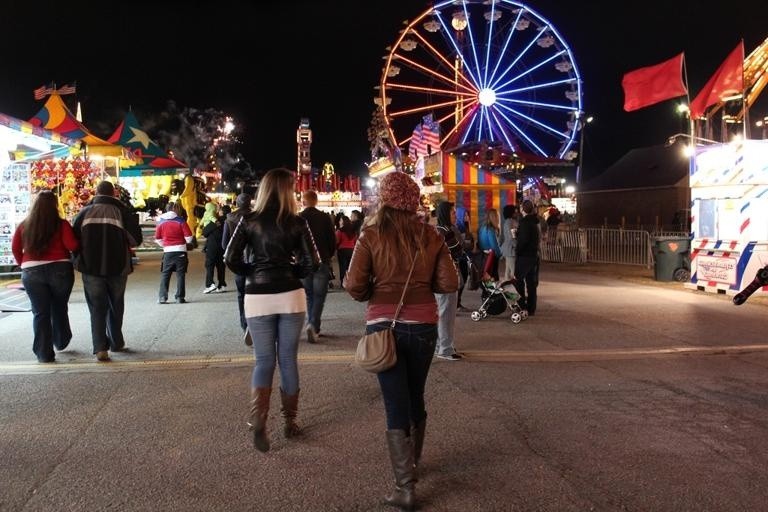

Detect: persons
[202,204,231,293]
[672,212,681,231]
[298,191,364,342]
[344,170,459,512]
[221,193,254,346]
[225,167,320,451]
[154,202,193,303]
[71,181,143,360]
[429,199,559,360]
[12,190,80,363]
[200,202,221,253]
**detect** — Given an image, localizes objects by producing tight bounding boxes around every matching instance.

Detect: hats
[236,193,252,208]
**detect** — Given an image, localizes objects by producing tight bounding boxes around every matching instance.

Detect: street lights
[575,110,594,191]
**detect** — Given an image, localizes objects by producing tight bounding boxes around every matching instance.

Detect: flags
[689,40,744,121]
[621,52,689,112]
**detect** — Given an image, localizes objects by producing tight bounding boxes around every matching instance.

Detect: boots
[247,387,273,452]
[277,385,301,438]
[385,411,427,508]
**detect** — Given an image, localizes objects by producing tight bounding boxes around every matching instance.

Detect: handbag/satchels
[322,261,335,280]
[356,327,398,373]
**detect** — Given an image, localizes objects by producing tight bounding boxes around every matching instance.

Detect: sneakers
[305,324,319,342]
[95,350,108,360]
[435,343,466,361]
[156,295,168,304]
[175,297,187,304]
[243,326,253,346]
[203,283,226,293]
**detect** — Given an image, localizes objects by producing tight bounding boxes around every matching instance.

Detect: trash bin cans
[655,236,691,283]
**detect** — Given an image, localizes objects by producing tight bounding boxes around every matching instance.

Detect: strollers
[462,248,529,325]
